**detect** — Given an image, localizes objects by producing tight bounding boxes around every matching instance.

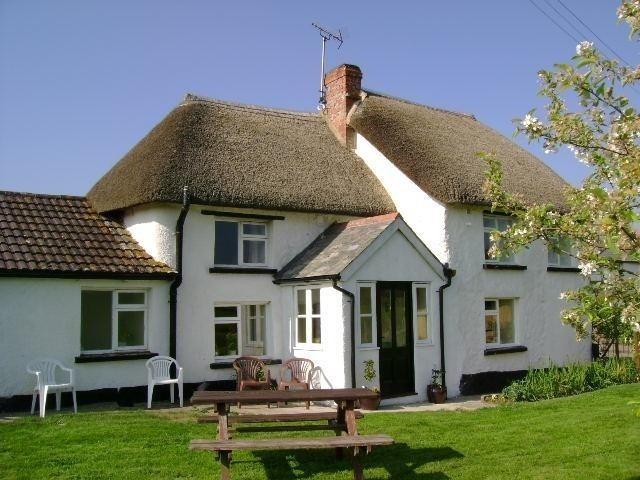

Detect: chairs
[233,357,270,408]
[26,357,77,417]
[277,358,314,409]
[145,355,183,408]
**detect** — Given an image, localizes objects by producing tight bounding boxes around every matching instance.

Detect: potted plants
[360,361,381,410]
[428,368,447,403]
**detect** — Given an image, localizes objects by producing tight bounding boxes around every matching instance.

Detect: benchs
[190,433,395,451]
[198,410,364,423]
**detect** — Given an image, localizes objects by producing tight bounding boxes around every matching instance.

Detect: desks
[190,386,378,480]
